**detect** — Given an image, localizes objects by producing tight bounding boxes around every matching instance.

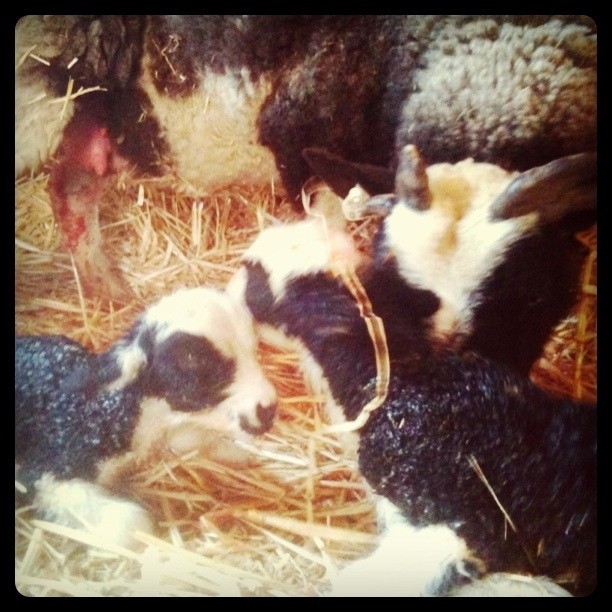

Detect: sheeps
[14,263,279,557]
[44,112,113,249]
[225,173,600,597]
[368,140,598,374]
[15,12,601,382]
